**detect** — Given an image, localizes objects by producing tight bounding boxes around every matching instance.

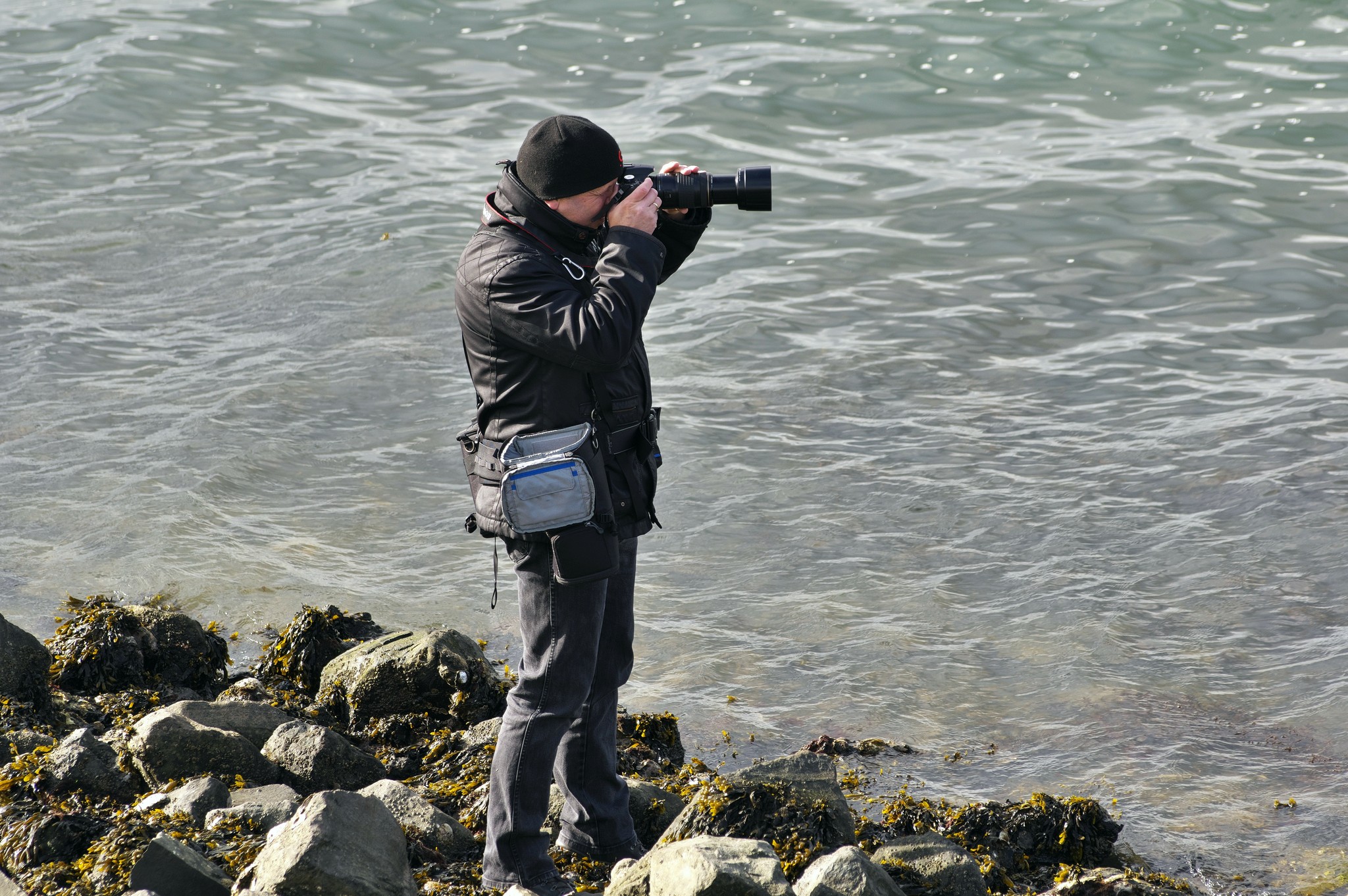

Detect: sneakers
[555,833,648,865]
[479,876,578,896]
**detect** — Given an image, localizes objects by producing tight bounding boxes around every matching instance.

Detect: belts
[608,411,659,455]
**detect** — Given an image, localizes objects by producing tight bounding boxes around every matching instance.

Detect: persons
[455,116,714,896]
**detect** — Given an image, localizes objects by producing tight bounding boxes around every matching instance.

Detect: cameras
[618,164,772,212]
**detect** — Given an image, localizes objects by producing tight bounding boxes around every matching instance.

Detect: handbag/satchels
[497,424,622,585]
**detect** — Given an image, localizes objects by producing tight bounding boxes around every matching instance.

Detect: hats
[516,115,625,201]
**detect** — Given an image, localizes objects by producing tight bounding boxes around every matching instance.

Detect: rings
[652,202,659,211]
